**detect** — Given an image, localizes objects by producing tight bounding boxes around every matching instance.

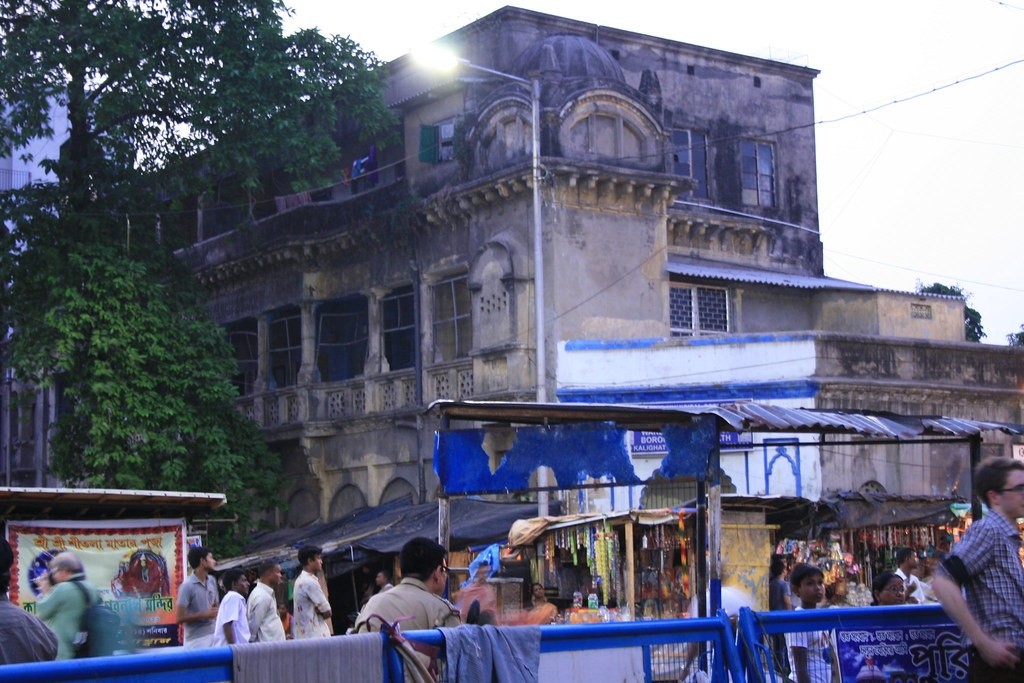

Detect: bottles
[587,593,598,609]
[572,591,582,608]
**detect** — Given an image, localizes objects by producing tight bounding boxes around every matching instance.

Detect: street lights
[414,45,550,516]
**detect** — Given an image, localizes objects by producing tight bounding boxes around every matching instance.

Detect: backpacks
[63,579,121,660]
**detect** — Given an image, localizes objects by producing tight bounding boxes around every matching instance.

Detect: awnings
[387,77,467,114]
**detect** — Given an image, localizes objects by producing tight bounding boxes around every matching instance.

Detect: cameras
[31,572,55,590]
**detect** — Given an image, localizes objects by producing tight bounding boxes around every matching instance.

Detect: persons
[355,536,462,635]
[175,547,220,649]
[277,601,290,634]
[870,548,926,606]
[247,558,286,643]
[527,583,558,624]
[0,538,58,664]
[677,569,756,683]
[932,456,1024,683]
[769,554,840,682]
[376,569,394,593]
[212,569,251,647]
[293,545,332,640]
[36,552,99,660]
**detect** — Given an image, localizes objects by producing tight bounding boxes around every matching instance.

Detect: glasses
[994,483,1024,496]
[441,565,451,576]
